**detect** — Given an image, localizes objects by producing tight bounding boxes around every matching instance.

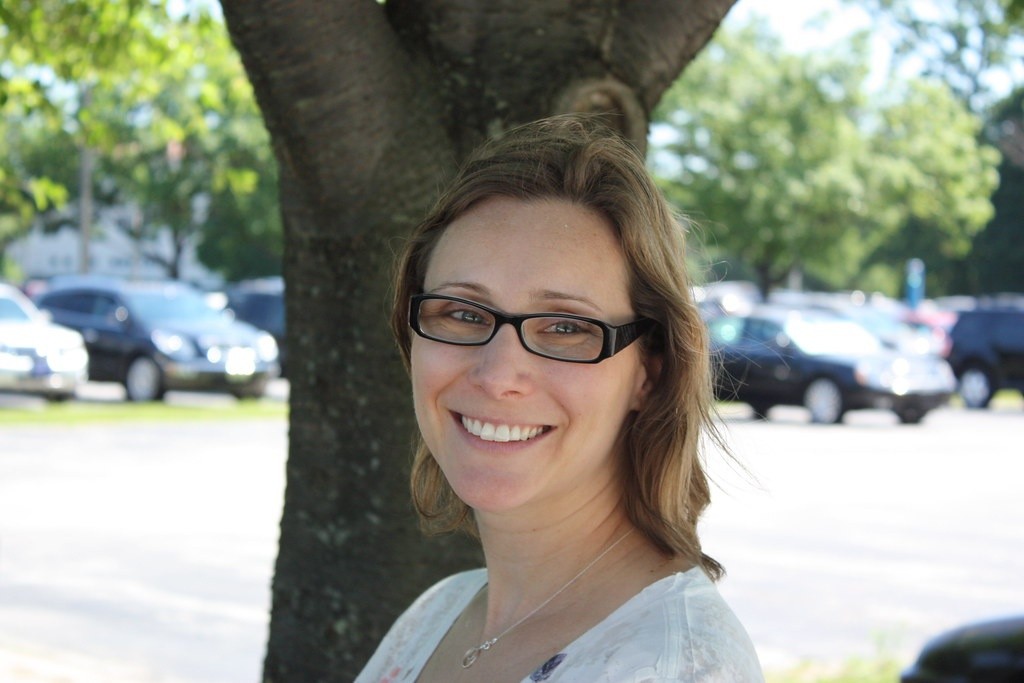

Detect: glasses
[406,292,654,365]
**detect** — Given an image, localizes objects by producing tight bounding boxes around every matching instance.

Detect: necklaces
[461,526,637,668]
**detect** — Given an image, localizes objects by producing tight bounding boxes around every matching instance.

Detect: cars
[946,294,1024,418]
[690,290,960,429]
[1,286,92,402]
[37,281,280,405]
[892,613,1024,683]
[230,274,286,368]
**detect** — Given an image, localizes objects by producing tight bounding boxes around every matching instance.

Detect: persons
[352,113,765,683]
